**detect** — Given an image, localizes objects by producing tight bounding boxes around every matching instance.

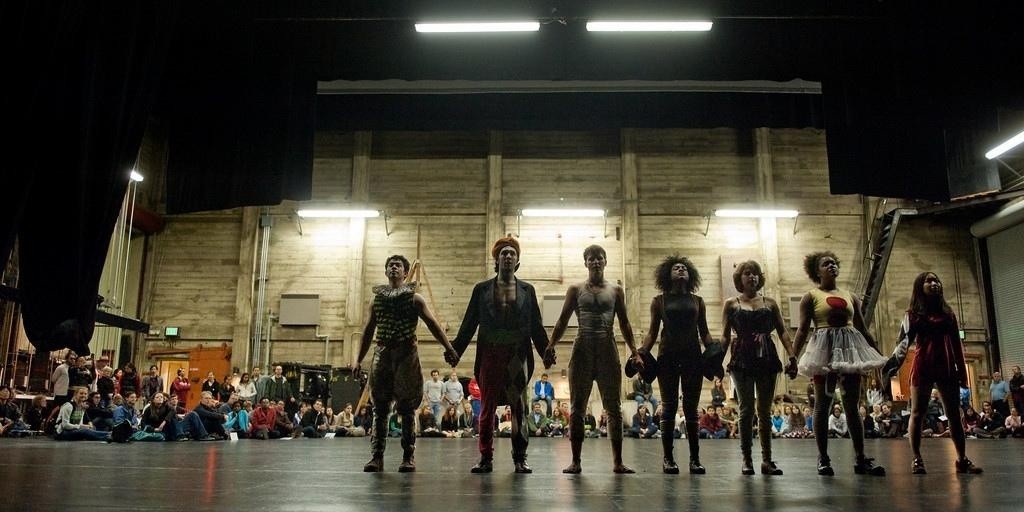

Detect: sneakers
[399,457,417,473]
[363,454,385,473]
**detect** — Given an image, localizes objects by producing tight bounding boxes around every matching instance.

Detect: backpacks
[44,401,77,434]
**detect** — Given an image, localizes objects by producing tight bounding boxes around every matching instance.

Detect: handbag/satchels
[112,419,133,443]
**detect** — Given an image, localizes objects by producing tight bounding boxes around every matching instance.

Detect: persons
[784,248,891,477]
[542,243,646,474]
[881,270,983,475]
[719,258,798,475]
[629,253,725,475]
[351,254,461,473]
[442,236,558,474]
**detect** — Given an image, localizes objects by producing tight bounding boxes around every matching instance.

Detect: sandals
[614,466,635,474]
[563,465,582,474]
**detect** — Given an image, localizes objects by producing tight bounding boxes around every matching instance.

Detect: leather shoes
[853,457,887,475]
[513,460,534,473]
[910,457,927,474]
[662,460,680,475]
[471,458,494,474]
[741,460,755,475]
[760,462,784,476]
[688,461,706,475]
[955,456,986,475]
[817,458,836,476]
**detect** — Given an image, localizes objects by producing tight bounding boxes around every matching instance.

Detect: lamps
[701,206,801,236]
[294,207,393,237]
[513,207,611,238]
